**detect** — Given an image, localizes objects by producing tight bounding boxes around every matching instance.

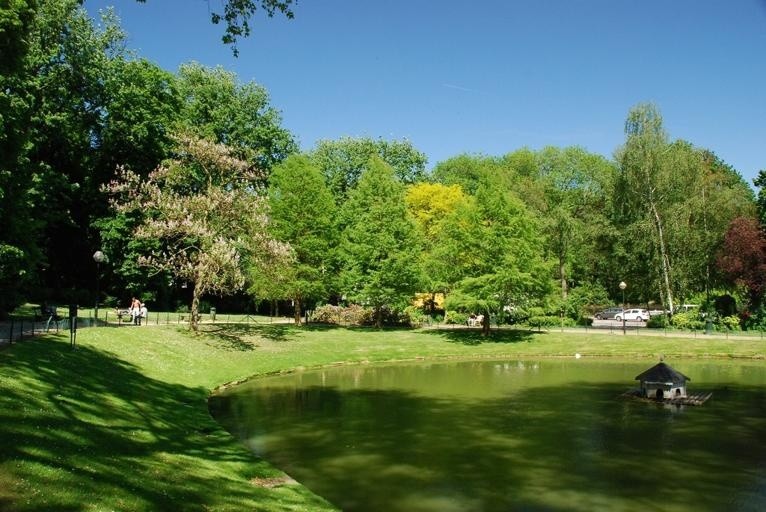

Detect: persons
[468,312,476,327]
[130,297,141,323]
[135,303,148,325]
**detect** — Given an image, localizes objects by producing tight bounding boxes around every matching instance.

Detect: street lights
[619,282,627,335]
[93,249,104,318]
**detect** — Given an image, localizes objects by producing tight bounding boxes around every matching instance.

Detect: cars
[594,308,650,322]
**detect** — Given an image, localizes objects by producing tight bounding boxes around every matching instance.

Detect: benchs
[32,306,64,324]
